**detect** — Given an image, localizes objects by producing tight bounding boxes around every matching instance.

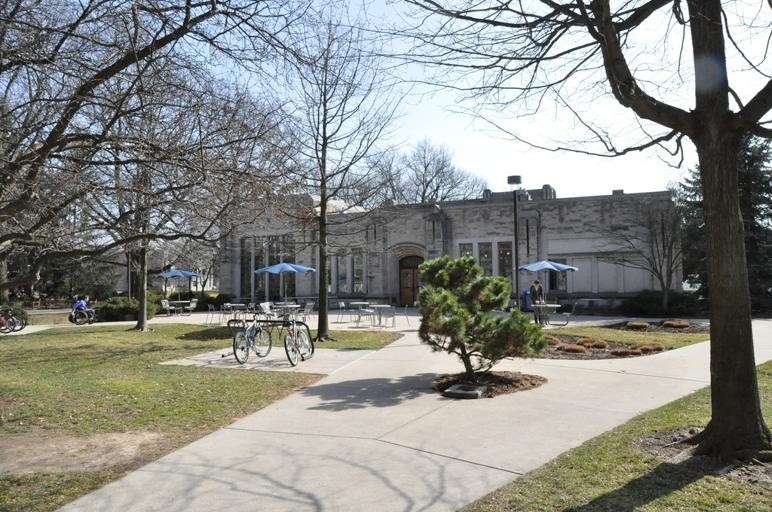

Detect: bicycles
[277,307,313,365]
[0,308,27,335]
[225,307,273,362]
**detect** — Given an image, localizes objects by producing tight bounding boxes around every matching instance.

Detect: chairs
[522,295,580,327]
[157,295,412,329]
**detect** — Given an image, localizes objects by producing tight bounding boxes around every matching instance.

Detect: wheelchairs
[68,307,98,325]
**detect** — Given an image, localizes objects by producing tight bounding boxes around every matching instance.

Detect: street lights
[506,172,524,318]
[428,200,441,257]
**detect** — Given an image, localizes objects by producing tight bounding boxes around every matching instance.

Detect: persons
[71,295,94,324]
[529,280,543,324]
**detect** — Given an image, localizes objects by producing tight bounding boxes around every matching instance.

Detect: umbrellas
[253,263,316,304]
[519,259,578,303]
[153,268,198,312]
